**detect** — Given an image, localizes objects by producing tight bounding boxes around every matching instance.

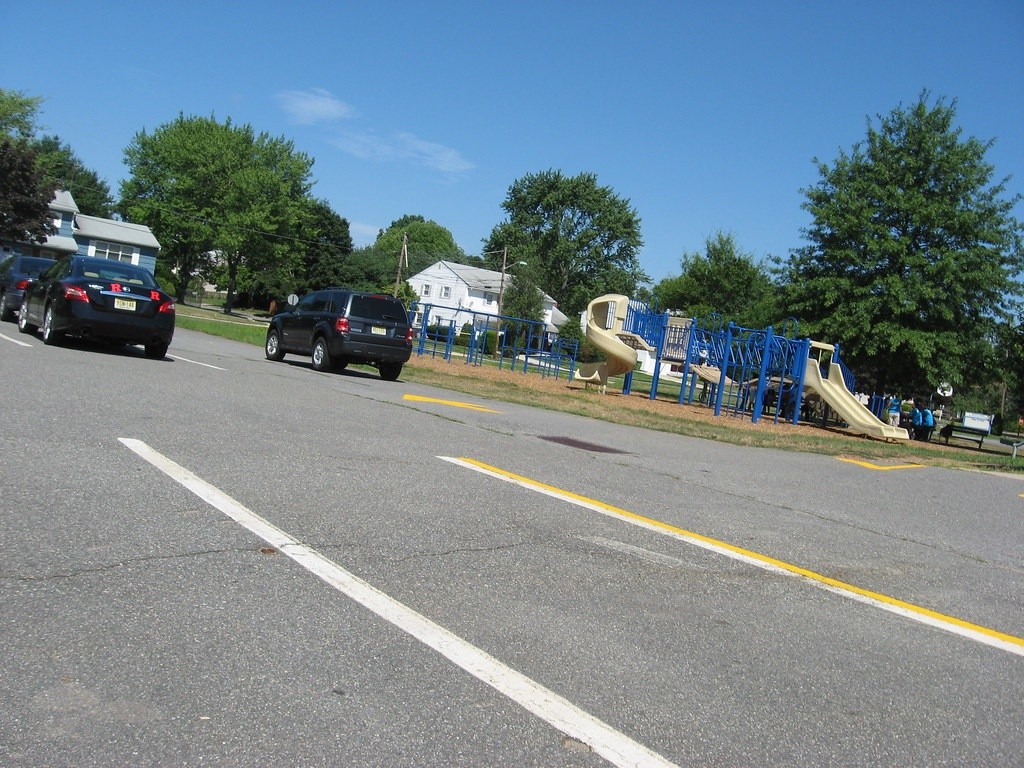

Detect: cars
[0,254,59,321]
[19,255,175,361]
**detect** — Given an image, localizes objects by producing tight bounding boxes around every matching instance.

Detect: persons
[889,393,902,426]
[739,385,936,441]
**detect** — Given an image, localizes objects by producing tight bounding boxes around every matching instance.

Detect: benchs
[937,411,995,450]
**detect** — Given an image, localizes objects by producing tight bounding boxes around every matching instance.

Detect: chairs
[900,413,913,439]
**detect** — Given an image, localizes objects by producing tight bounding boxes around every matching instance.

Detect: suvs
[264,287,413,381]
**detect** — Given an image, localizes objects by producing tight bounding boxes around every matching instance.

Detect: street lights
[493,260,527,361]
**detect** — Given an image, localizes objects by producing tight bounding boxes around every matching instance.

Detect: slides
[804,358,910,440]
[574,294,638,385]
